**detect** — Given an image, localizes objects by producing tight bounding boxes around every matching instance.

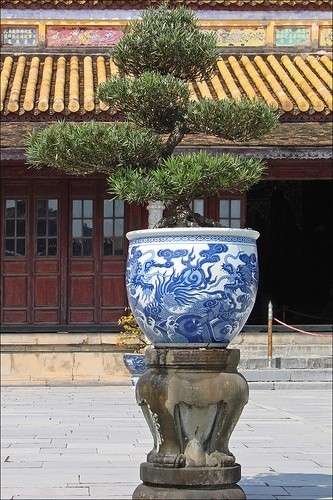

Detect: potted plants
[108,312,147,388]
[22,3,277,349]
